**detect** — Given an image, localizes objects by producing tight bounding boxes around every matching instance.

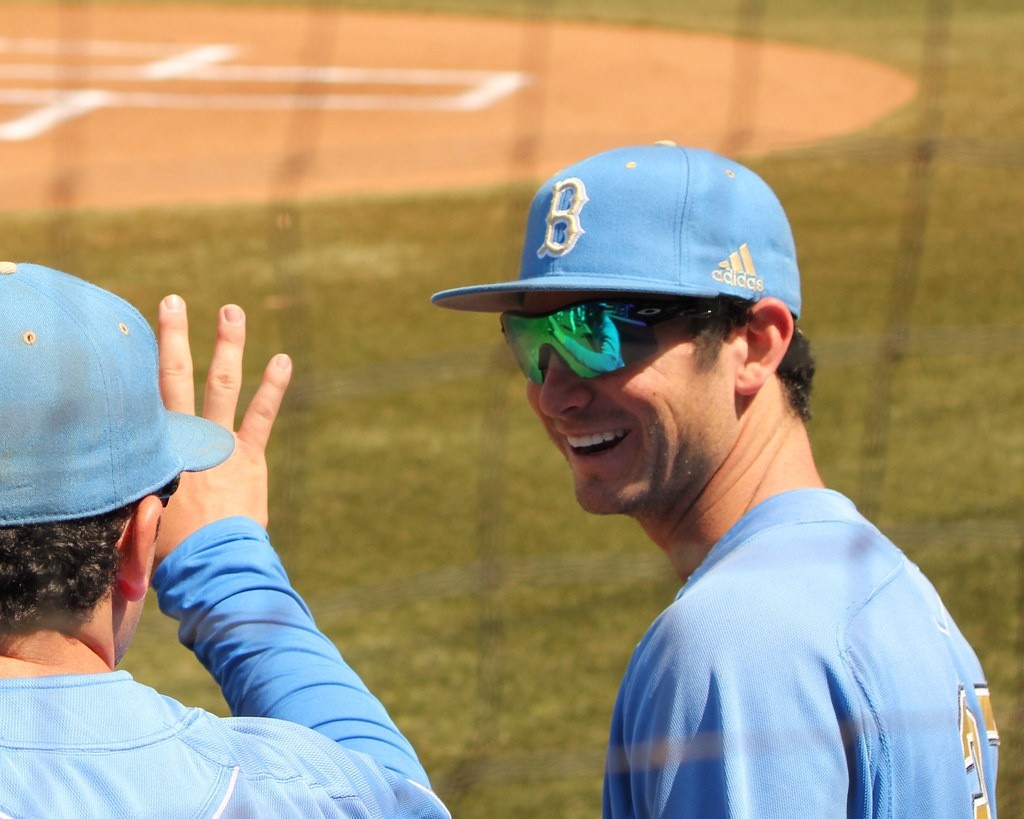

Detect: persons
[0,258,450,819]
[429,139,1002,819]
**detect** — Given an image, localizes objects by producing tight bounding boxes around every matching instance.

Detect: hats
[0,257,236,526]
[429,137,803,323]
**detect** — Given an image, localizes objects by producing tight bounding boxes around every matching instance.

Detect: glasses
[500,302,716,381]
